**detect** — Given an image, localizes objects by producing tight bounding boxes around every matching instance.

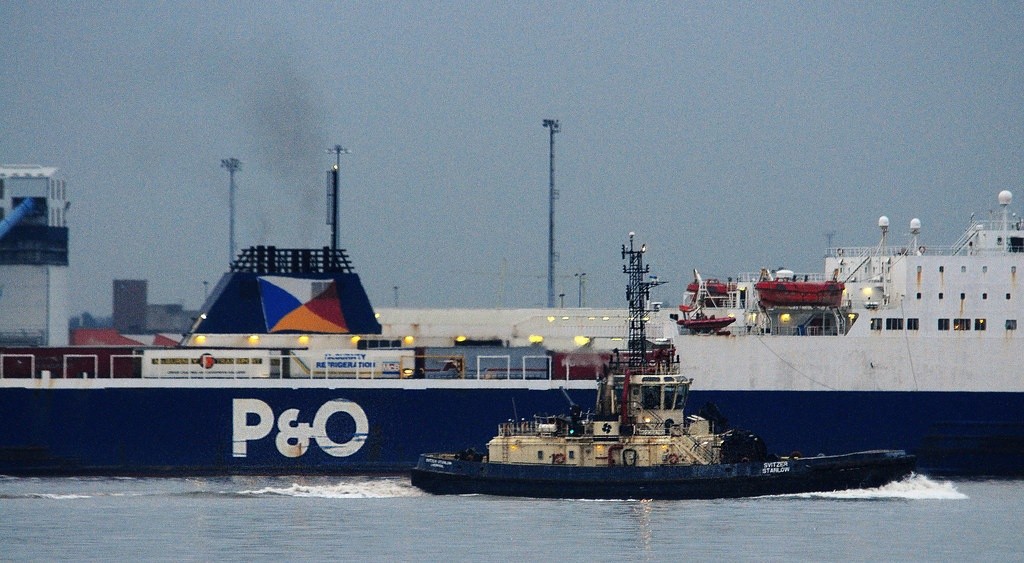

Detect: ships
[0,188,1023,477]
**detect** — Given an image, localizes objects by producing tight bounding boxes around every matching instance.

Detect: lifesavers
[669,454,679,465]
[555,453,565,465]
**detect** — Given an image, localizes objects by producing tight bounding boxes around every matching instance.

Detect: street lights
[541,117,561,308]
[219,156,242,267]
[559,292,565,309]
[573,273,588,307]
[324,144,352,266]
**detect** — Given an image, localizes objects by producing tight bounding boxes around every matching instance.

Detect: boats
[408,231,915,500]
[755,278,845,307]
[677,316,736,332]
